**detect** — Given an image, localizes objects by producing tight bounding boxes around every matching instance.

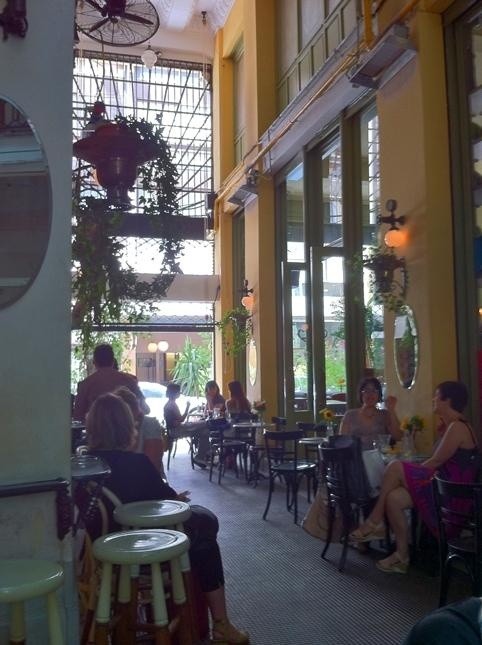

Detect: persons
[217,379,252,470]
[162,383,210,469]
[73,343,151,425]
[336,374,403,554]
[71,392,254,643]
[114,384,169,480]
[202,380,227,416]
[347,379,481,575]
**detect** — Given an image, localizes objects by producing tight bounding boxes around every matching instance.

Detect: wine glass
[367,432,419,465]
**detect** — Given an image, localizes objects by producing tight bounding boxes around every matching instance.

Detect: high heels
[375,551,409,573]
[349,520,386,542]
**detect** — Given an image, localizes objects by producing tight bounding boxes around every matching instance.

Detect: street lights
[147,343,157,382]
[157,341,169,383]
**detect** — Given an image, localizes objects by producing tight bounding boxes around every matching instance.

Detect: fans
[74,1,160,48]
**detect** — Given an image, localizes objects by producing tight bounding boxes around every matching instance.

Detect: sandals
[213,618,248,644]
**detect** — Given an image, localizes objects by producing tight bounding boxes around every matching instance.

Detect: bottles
[197,403,222,419]
[73,428,89,456]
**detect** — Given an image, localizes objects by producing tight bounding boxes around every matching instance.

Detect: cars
[137,381,210,426]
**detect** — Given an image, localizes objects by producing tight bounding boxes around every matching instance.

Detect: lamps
[71,118,157,213]
[361,199,406,293]
[141,36,159,70]
[237,279,254,308]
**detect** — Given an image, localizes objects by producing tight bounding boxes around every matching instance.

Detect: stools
[0,499,198,645]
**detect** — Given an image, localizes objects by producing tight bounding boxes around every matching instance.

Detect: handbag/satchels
[362,448,387,498]
[301,494,343,543]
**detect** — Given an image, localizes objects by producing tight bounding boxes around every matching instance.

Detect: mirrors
[393,304,419,390]
[0,96,53,311]
[246,340,259,386]
